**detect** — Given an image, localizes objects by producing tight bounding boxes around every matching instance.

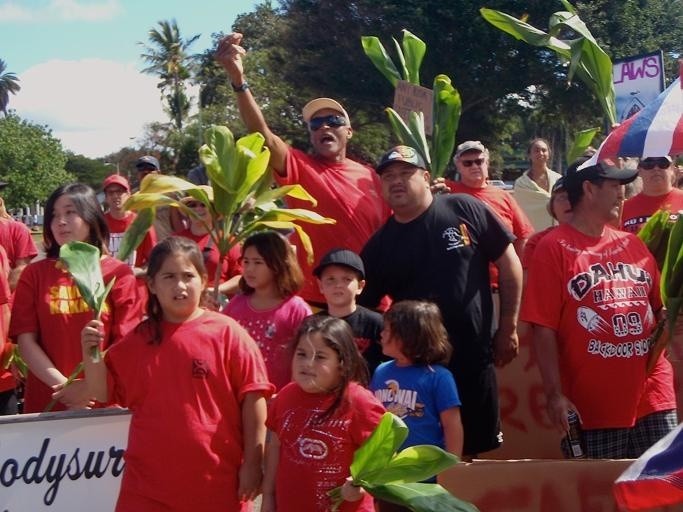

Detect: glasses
[107,189,126,196]
[309,114,346,132]
[137,164,156,172]
[642,158,671,170]
[188,202,206,207]
[464,159,485,166]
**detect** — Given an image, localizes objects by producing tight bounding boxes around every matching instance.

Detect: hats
[102,174,131,192]
[301,96,346,122]
[456,141,485,155]
[137,155,161,173]
[375,145,427,171]
[552,178,564,192]
[178,191,212,217]
[313,250,366,280]
[565,157,641,190]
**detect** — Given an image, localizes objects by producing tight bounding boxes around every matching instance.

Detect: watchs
[231,82,249,93]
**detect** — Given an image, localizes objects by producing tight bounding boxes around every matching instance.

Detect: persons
[314,248,396,387]
[618,156,683,235]
[80,236,277,512]
[215,30,452,311]
[521,176,573,290]
[169,185,244,301]
[186,143,256,235]
[0,244,10,281]
[7,182,142,414]
[583,145,596,156]
[199,232,314,394]
[518,158,678,460]
[513,138,563,233]
[131,156,190,244]
[443,140,534,336]
[0,261,13,416]
[0,198,38,311]
[260,315,389,511]
[369,299,464,512]
[618,157,644,200]
[355,145,523,463]
[102,174,157,321]
